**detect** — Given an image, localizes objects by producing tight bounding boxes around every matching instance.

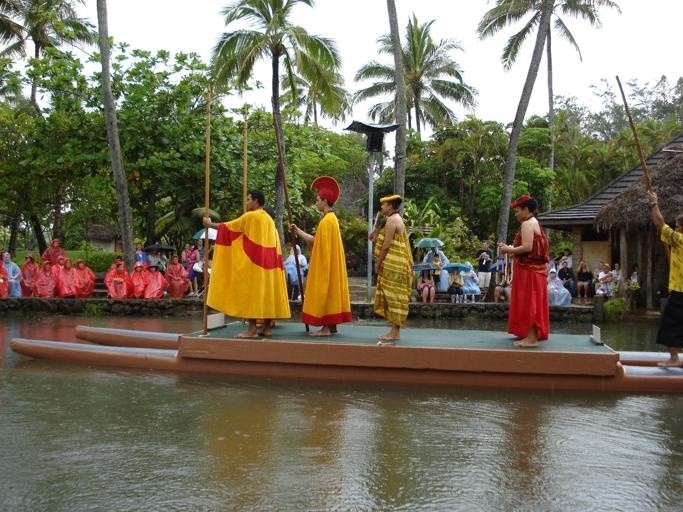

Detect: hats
[311,176,340,205]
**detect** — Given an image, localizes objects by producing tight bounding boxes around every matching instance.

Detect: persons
[0,238,212,300]
[646,189,683,367]
[373,195,414,340]
[287,172,353,336]
[498,193,551,347]
[202,190,292,338]
[283,244,307,300]
[415,242,638,307]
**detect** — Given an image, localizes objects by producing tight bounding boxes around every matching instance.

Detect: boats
[12,319,683,396]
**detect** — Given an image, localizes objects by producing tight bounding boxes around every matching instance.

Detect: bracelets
[648,203,657,209]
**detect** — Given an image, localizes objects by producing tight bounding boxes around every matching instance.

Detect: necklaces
[322,210,336,219]
[526,216,533,220]
[389,211,398,216]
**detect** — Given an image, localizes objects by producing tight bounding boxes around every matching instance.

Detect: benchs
[90,272,108,298]
[414,284,596,303]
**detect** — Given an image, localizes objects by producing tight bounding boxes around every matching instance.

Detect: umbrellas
[192,228,218,240]
[413,238,445,249]
